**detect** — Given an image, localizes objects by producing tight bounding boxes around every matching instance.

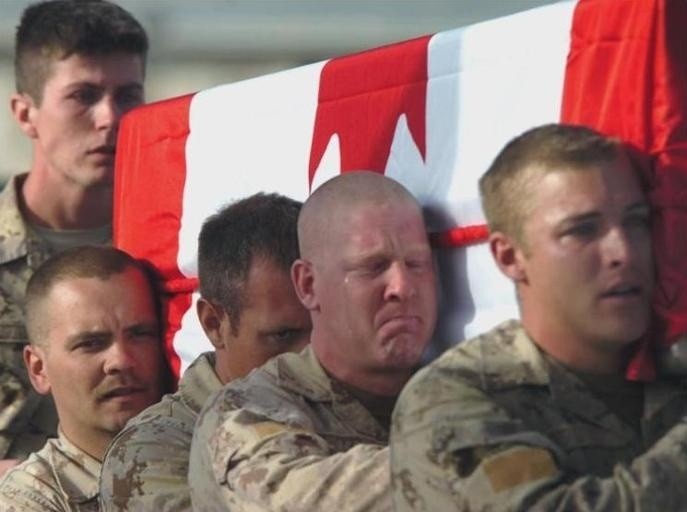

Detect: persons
[1,247,161,512]
[188,170,437,512]
[391,123,686,512]
[0,0,149,482]
[101,192,312,512]
